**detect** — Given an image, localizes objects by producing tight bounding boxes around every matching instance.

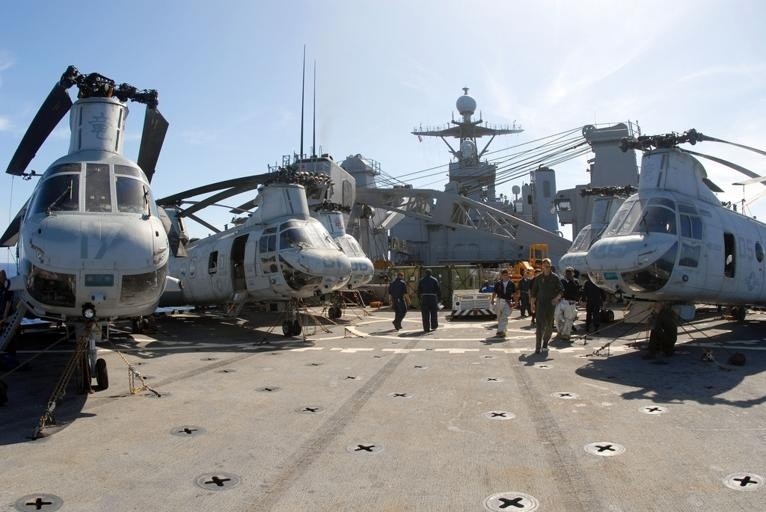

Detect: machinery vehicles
[506,244,548,307]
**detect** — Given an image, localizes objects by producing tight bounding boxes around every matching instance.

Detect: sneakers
[551,332,570,340]
[495,330,507,338]
[392,320,403,331]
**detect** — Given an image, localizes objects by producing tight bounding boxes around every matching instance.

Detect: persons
[0,269,13,321]
[389,272,411,330]
[530,257,565,354]
[550,265,558,329]
[645,299,680,361]
[417,269,442,333]
[479,280,495,293]
[582,281,606,332]
[570,269,582,332]
[555,267,580,340]
[527,269,542,326]
[517,271,534,317]
[490,270,517,338]
